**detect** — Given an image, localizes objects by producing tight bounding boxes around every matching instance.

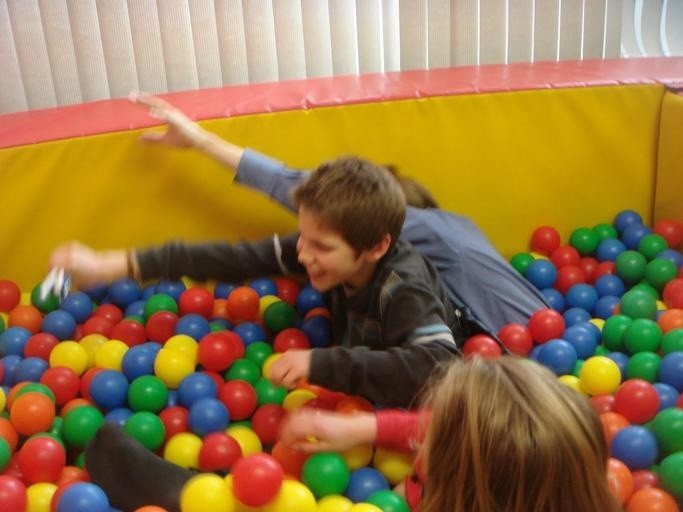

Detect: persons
[46,154,464,511]
[127,88,556,358]
[403,352,620,511]
